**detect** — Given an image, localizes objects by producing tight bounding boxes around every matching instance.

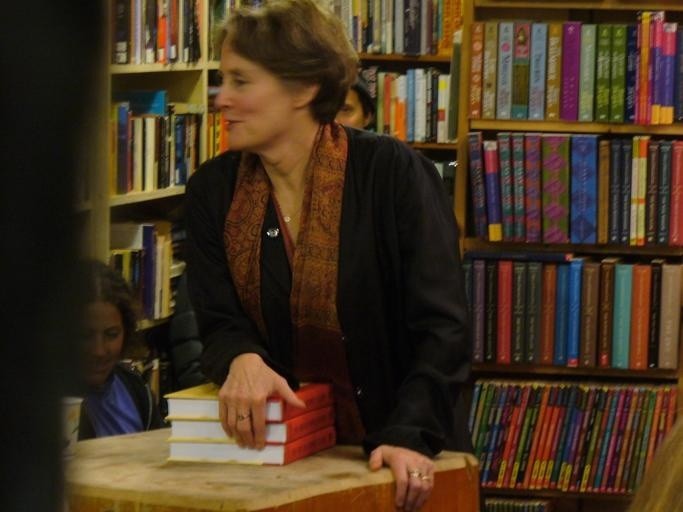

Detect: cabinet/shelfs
[80,1,683,512]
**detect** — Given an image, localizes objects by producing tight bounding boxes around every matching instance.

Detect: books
[114,1,464,70]
[164,404,335,445]
[467,132,683,247]
[112,72,233,327]
[464,252,683,369]
[467,10,682,125]
[163,423,336,467]
[361,65,459,188]
[161,378,333,424]
[468,380,682,511]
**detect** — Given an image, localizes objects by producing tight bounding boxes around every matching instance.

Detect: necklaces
[281,202,303,225]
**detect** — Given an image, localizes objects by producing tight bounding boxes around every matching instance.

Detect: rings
[236,413,250,421]
[407,467,422,479]
[420,475,434,483]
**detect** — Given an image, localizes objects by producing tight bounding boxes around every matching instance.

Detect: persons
[330,76,375,131]
[65,252,163,441]
[150,1,489,512]
